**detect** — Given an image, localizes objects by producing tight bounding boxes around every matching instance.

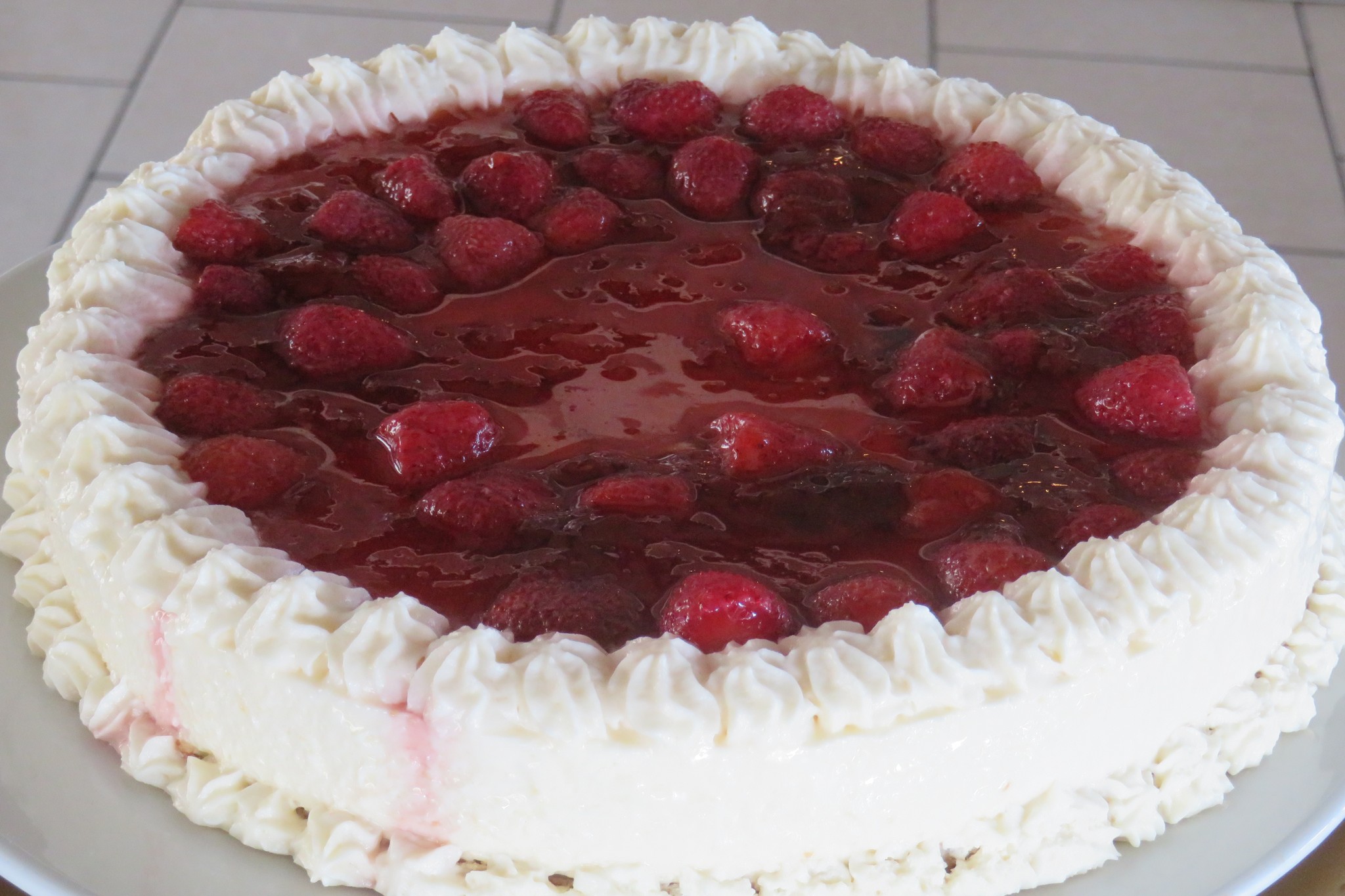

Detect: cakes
[0,13,1345,896]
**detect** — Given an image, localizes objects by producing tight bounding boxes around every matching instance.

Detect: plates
[0,207,1345,896]
